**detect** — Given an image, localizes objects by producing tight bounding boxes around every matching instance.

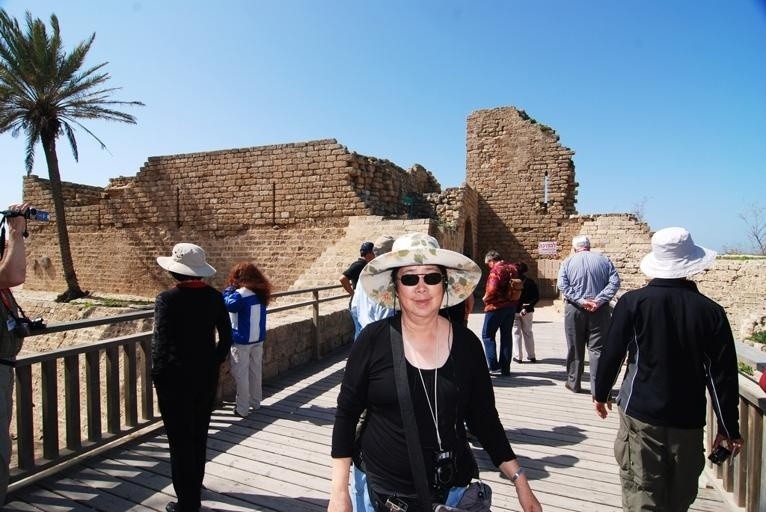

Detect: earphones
[443,276,448,289]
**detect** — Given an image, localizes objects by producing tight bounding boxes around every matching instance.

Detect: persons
[512,262,541,363]
[556,234,622,403]
[480,249,525,379]
[222,260,274,418]
[339,241,376,343]
[593,226,746,511]
[326,230,543,512]
[150,241,235,511]
[351,233,399,338]
[0,199,33,510]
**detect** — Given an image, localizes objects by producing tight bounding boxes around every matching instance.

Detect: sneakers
[513,358,522,363]
[488,367,502,375]
[527,357,535,362]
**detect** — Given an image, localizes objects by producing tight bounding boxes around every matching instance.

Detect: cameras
[25,206,51,222]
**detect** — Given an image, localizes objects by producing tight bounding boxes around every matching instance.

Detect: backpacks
[505,278,523,302]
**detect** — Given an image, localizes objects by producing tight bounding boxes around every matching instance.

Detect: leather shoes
[167,501,177,511]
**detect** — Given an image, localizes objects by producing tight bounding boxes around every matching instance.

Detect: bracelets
[510,466,525,482]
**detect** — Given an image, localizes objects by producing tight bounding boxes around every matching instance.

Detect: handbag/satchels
[432,482,492,512]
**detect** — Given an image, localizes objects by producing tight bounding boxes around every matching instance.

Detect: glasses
[398,271,442,286]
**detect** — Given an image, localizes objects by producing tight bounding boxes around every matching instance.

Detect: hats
[571,236,589,249]
[640,227,718,279]
[157,243,216,277]
[359,233,483,312]
